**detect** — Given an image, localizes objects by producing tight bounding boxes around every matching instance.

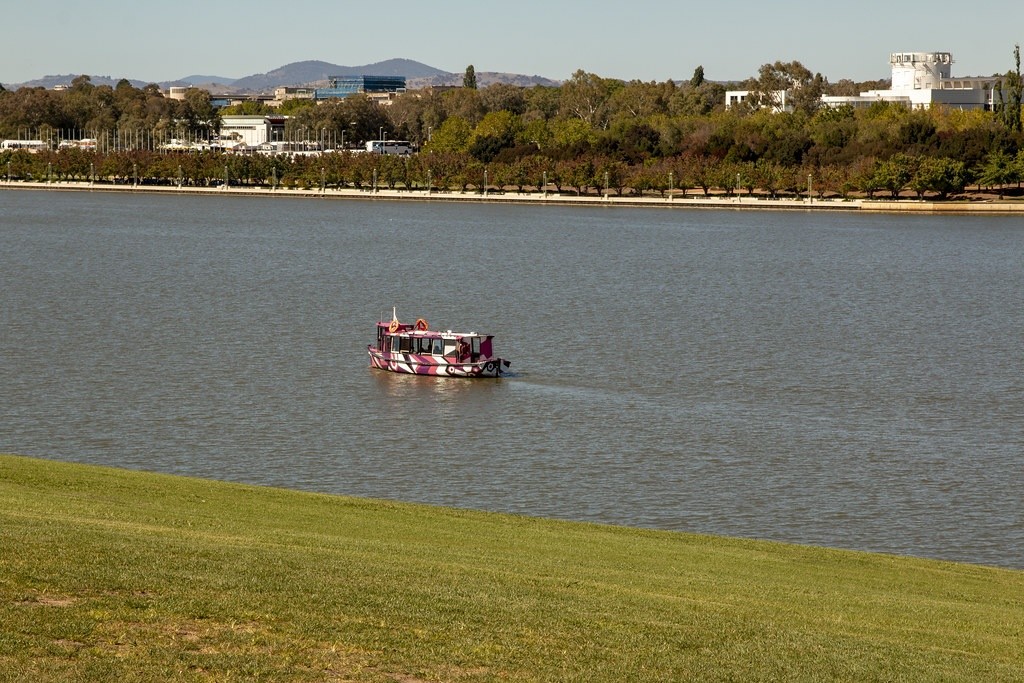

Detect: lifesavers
[388,320,400,333]
[416,319,428,331]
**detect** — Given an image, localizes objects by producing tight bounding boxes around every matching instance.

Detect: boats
[368,307,511,378]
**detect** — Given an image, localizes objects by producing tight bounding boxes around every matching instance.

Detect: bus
[366,140,414,154]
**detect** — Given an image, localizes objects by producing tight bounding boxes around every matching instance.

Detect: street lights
[736,173,741,203]
[8,162,11,184]
[379,126,384,155]
[47,161,54,188]
[483,170,488,196]
[427,169,433,196]
[807,174,813,206]
[321,168,326,192]
[372,168,378,193]
[272,166,277,190]
[383,131,388,155]
[542,170,547,197]
[134,164,138,187]
[224,164,229,189]
[178,165,182,189]
[669,172,673,202]
[341,129,346,150]
[274,125,331,153]
[90,162,95,187]
[604,171,609,201]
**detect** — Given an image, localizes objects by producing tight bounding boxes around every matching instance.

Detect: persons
[460,341,470,354]
[421,344,439,353]
